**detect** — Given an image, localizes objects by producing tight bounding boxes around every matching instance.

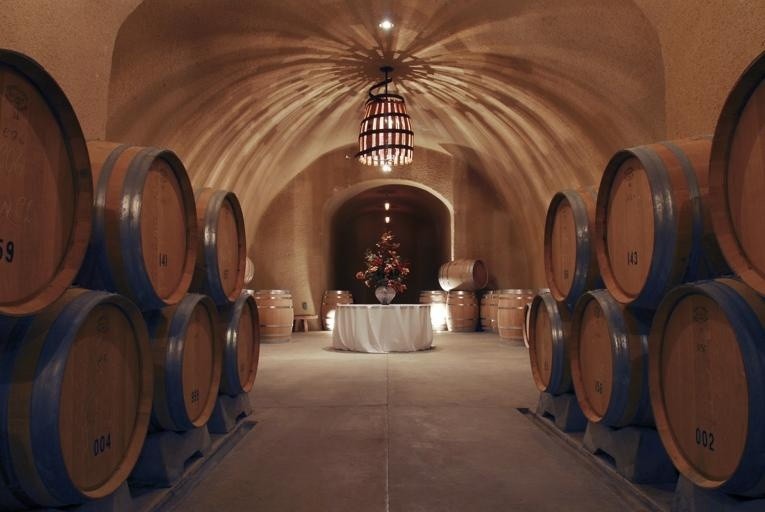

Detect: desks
[331,303,433,353]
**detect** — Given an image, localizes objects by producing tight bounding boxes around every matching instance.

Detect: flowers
[355,231,409,293]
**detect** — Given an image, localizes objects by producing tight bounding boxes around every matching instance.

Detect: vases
[374,286,396,304]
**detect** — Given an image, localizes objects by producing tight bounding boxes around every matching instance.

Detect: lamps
[354,65,414,172]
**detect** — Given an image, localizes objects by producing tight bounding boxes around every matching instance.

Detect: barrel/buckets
[136,292,223,433]
[593,135,733,311]
[240,289,255,296]
[70,141,198,313]
[569,288,657,429]
[544,190,607,303]
[480,291,491,332]
[488,291,499,333]
[253,290,294,344]
[438,259,488,291]
[217,296,260,398]
[529,293,576,397]
[522,303,533,349]
[1,285,155,512]
[420,291,447,331]
[446,290,479,332]
[187,188,247,307]
[1,49,93,318]
[648,273,765,500]
[707,49,765,298]
[322,290,353,331]
[497,289,537,339]
